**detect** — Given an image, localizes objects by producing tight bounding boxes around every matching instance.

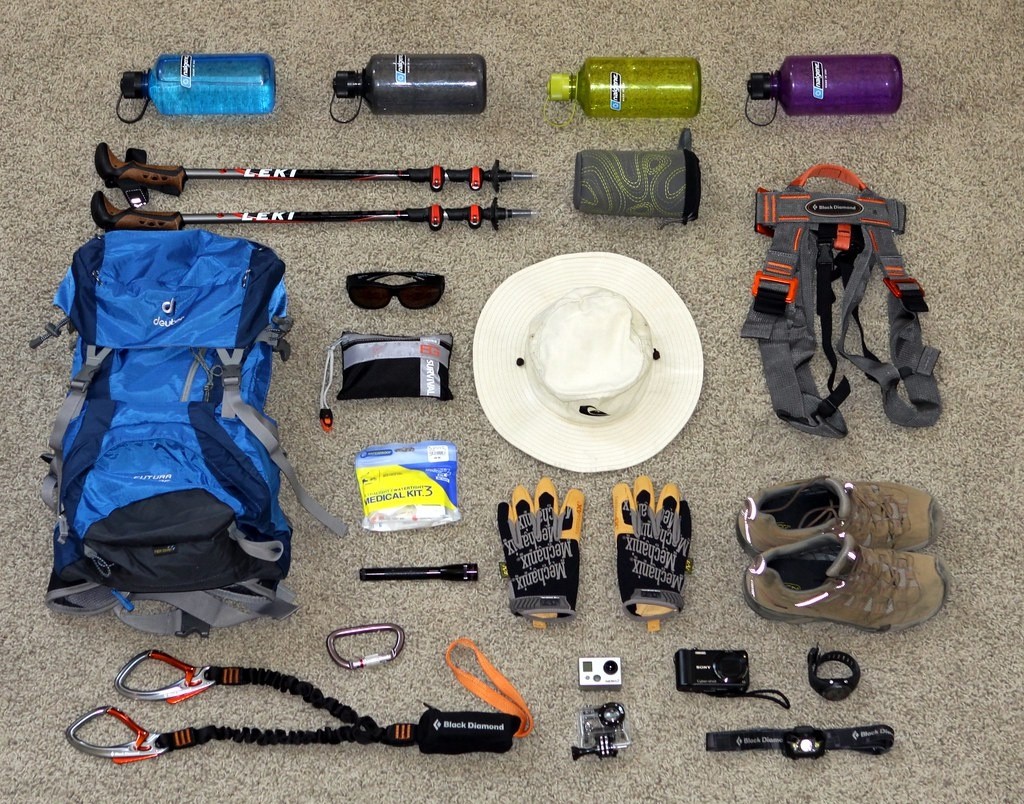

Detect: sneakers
[736,476,949,634]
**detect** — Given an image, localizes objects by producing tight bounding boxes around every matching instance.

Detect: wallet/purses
[320,331,455,432]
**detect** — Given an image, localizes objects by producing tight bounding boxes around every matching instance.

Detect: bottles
[745,54,902,127]
[115,53,276,124]
[329,53,486,123]
[542,55,703,127]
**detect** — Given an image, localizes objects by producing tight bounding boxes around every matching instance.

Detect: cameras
[675,648,750,694]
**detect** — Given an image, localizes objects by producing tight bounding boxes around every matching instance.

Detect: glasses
[346,271,446,310]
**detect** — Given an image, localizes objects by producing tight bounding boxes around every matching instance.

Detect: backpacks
[29,230,350,639]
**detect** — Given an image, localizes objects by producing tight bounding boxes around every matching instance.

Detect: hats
[472,251,704,473]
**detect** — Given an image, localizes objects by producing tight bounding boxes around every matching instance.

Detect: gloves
[496,478,585,630]
[612,475,693,632]
